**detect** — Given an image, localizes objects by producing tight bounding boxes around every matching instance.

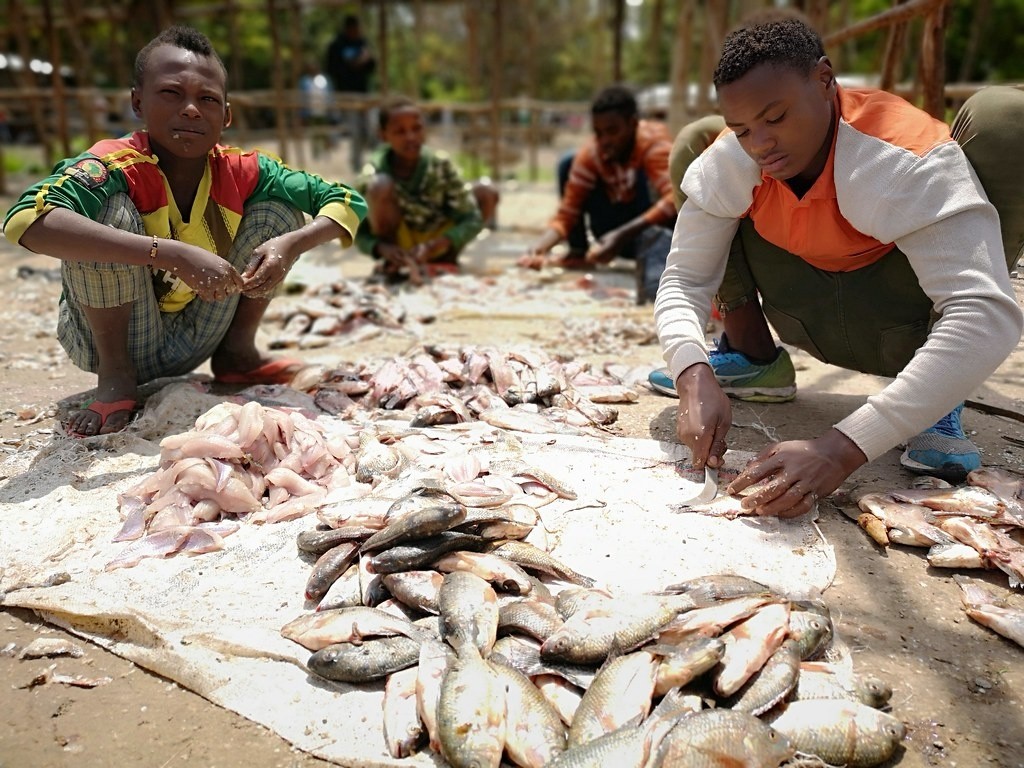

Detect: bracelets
[151,233,160,264]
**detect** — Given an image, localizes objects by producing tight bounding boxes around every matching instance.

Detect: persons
[523,86,678,273]
[321,15,378,134]
[4,26,369,432]
[648,19,1024,517]
[346,94,500,279]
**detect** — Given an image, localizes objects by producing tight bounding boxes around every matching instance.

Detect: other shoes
[549,251,593,268]
[370,264,395,285]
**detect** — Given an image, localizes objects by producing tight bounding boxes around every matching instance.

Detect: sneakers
[898,402,981,480]
[647,331,796,403]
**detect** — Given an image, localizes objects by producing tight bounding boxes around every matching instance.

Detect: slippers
[216,360,301,383]
[65,399,136,438]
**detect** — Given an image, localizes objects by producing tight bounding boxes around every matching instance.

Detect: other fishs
[115,342,1024,768]
